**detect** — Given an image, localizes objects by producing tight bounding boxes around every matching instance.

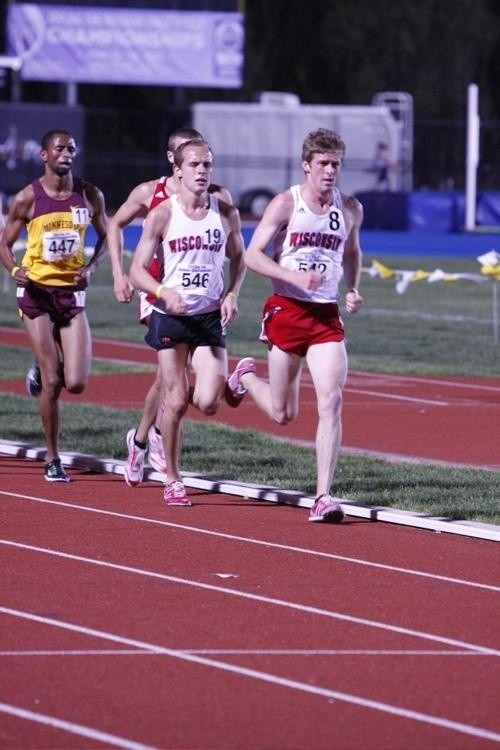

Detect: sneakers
[26,362,42,397]
[225,356,256,408]
[44,457,70,482]
[124,422,191,506]
[309,494,344,523]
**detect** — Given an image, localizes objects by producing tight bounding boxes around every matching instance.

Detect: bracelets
[156,285,164,299]
[227,292,237,298]
[347,287,358,294]
[11,266,20,277]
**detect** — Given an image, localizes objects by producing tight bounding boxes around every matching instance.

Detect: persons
[224,125,364,524]
[369,143,401,192]
[0,129,108,482]
[106,126,232,487]
[128,139,247,508]
